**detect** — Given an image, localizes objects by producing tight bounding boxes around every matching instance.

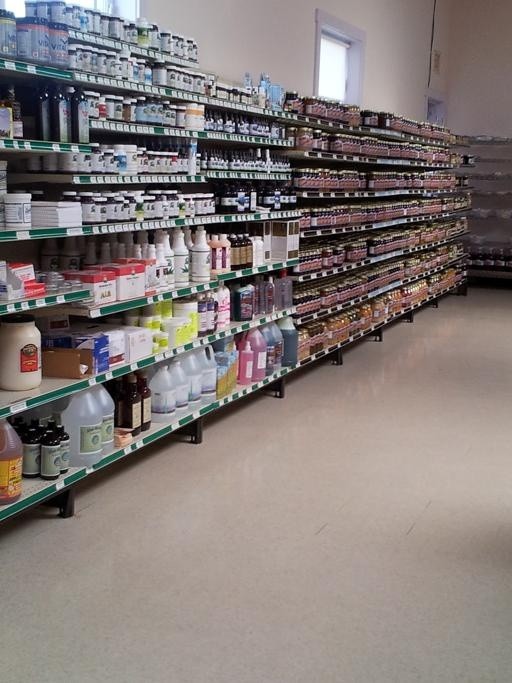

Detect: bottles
[0,0,512,506]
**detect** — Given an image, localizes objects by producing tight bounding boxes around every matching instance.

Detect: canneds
[0,0,475,362]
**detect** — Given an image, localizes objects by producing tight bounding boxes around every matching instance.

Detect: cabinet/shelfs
[0,56,512,527]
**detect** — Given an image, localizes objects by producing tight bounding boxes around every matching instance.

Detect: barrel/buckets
[149,345,217,424]
[61,384,115,467]
[0,314,42,389]
[238,317,299,382]
